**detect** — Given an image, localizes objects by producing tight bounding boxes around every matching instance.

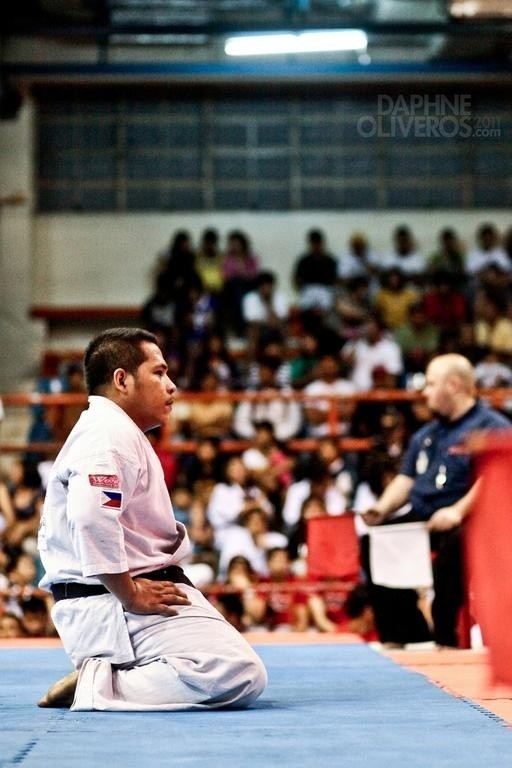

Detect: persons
[37,328,266,712]
[1,221,511,650]
